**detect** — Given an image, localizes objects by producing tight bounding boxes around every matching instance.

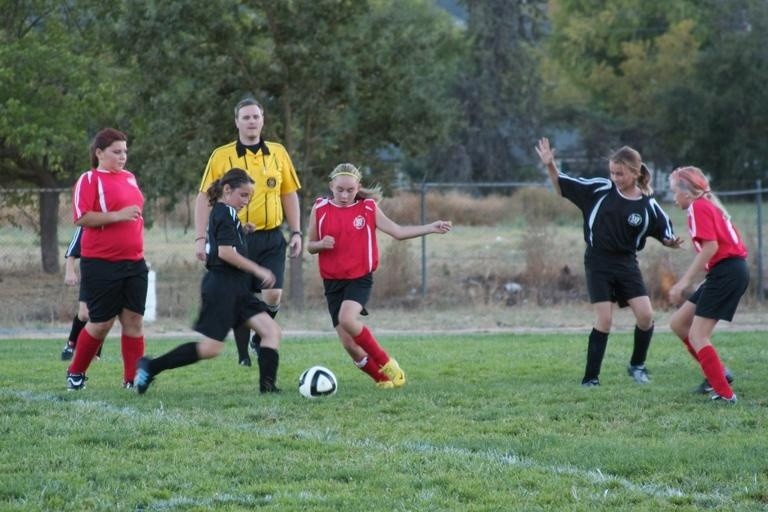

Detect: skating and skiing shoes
[65,374,88,391]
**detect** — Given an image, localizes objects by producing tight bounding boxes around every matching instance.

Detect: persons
[535,137,685,389]
[668,166,750,405]
[133,168,283,396]
[61,225,101,360]
[65,128,148,393]
[306,162,452,391]
[193,98,302,367]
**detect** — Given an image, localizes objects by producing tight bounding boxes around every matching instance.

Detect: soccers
[299,366,337,399]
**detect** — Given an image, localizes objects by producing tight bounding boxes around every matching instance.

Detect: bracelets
[292,231,302,238]
[195,237,206,242]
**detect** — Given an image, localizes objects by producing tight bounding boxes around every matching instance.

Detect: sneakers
[133,355,153,395]
[698,370,734,394]
[579,378,604,389]
[378,357,406,389]
[375,379,394,390]
[61,341,73,361]
[627,362,654,388]
[712,393,738,408]
